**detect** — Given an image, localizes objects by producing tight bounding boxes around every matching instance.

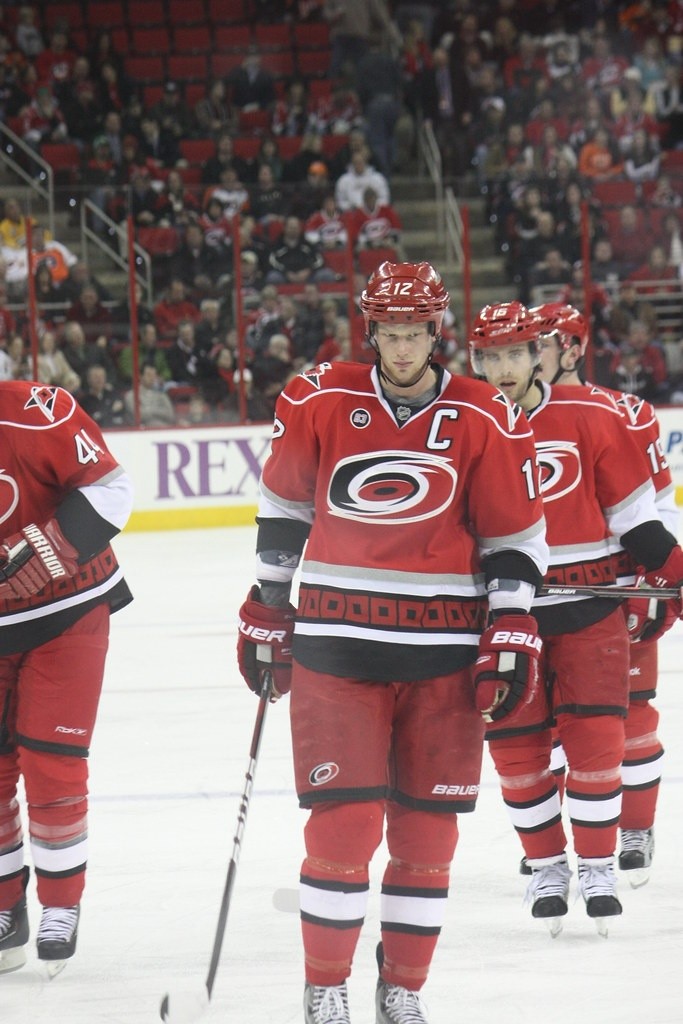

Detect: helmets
[360,258,448,337]
[468,302,542,349]
[529,302,590,356]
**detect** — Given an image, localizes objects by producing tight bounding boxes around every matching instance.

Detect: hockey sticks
[534,584,682,603]
[158,666,275,1024]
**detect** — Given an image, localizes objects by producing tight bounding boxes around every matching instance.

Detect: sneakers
[525,859,571,937]
[520,857,535,875]
[577,855,623,938]
[303,981,351,1024]
[374,941,428,1024]
[0,865,30,976]
[618,827,654,889]
[37,903,79,980]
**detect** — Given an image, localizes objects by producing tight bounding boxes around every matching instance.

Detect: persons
[0,0,683,430]
[0,381,134,980]
[470,300,683,937]
[235,261,552,1024]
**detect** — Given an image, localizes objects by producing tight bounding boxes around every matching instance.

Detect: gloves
[471,614,544,728]
[624,566,679,648]
[235,584,296,703]
[642,545,682,621]
[0,518,81,600]
